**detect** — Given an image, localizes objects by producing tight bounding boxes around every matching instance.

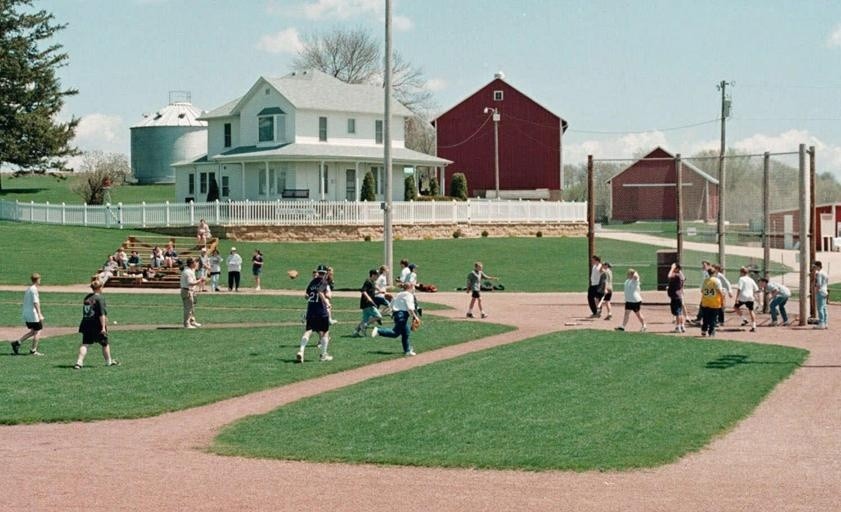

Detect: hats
[408,264,420,271]
[316,265,329,275]
[370,269,382,276]
[186,255,200,263]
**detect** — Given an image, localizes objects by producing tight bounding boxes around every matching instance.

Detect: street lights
[484,106,501,197]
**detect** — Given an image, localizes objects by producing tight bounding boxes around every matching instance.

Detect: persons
[207,249,223,292]
[301,272,337,348]
[327,266,336,288]
[689,260,710,326]
[295,266,333,363]
[667,275,685,333]
[732,266,760,332]
[88,237,177,288]
[586,256,604,318]
[669,263,691,323]
[396,258,410,292]
[466,262,499,319]
[710,264,734,328]
[74,281,120,369]
[372,283,420,357]
[700,267,724,337]
[352,269,382,338]
[404,264,423,321]
[251,249,265,292]
[374,266,396,326]
[226,246,242,292]
[11,273,45,356]
[179,257,206,330]
[614,268,647,332]
[198,248,210,292]
[197,219,211,253]
[101,175,112,207]
[595,262,613,320]
[757,278,792,326]
[812,261,830,329]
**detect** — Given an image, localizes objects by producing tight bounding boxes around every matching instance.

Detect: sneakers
[614,323,647,334]
[184,321,202,331]
[106,362,119,368]
[590,310,614,321]
[296,351,303,366]
[318,352,335,362]
[353,321,369,338]
[11,340,22,356]
[741,318,757,334]
[73,363,86,371]
[371,327,379,337]
[406,350,416,357]
[30,348,46,356]
[671,326,685,332]
[770,320,792,326]
[467,312,489,319]
